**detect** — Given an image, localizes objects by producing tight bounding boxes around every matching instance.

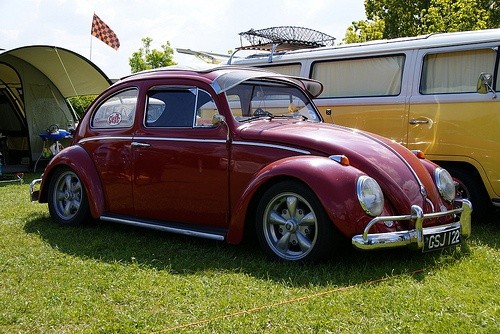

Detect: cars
[29,65,473,266]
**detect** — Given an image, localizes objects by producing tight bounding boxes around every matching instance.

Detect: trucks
[199,26,500,226]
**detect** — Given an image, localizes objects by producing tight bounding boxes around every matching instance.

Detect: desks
[34,135,73,173]
